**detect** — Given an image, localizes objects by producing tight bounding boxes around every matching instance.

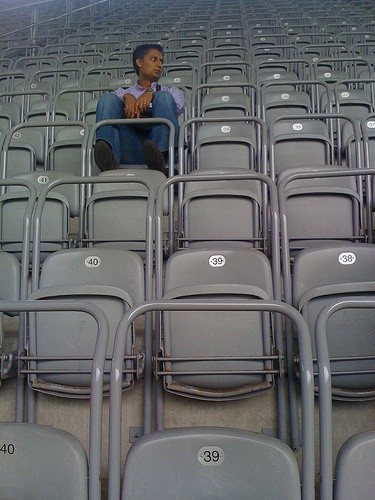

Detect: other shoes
[94,139,116,172]
[144,140,164,172]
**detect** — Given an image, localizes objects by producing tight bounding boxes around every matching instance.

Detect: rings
[143,103,146,105]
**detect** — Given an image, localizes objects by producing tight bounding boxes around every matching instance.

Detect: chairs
[1,0,375,500]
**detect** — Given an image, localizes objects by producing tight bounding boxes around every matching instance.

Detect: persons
[94,43,185,186]
